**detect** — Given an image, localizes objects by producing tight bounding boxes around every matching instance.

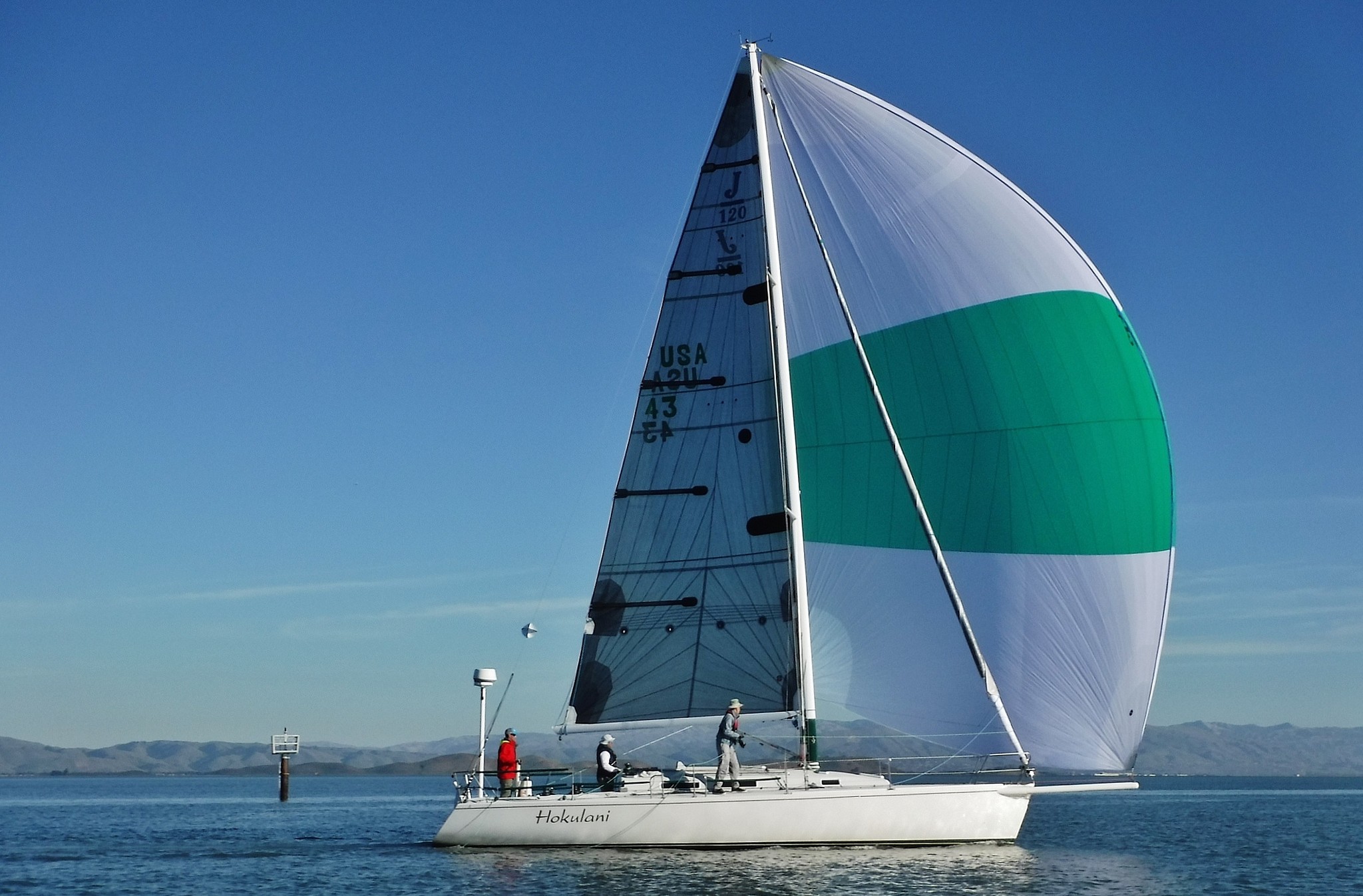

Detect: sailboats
[428,43,1176,844]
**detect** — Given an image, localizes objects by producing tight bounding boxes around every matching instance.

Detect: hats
[727,699,744,709]
[505,728,522,735]
[599,734,615,745]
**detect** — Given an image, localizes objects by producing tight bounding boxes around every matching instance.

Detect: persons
[497,728,521,800]
[594,734,623,792]
[712,698,749,795]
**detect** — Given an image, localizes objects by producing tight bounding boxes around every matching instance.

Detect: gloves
[736,730,747,738]
[738,739,746,748]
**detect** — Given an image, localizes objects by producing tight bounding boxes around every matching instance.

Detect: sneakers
[712,789,726,795]
[731,788,748,793]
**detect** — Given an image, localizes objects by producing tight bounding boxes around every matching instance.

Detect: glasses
[509,733,516,736]
